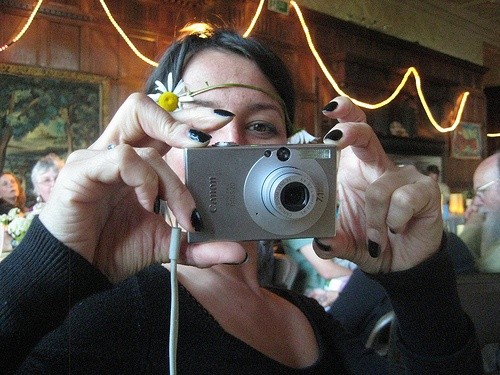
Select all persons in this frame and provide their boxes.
[458,153,500,273]
[0,171,31,215]
[257,238,358,314]
[31,153,65,202]
[0,28,485,375]
[425,165,451,205]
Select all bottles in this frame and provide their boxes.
[465,192,473,208]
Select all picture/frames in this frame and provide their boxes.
[0,63,111,214]
[449,119,485,161]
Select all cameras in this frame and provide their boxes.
[183,143,337,244]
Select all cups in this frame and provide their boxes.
[449,193,464,215]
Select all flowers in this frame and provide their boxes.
[147,72,193,113]
[0,202,45,248]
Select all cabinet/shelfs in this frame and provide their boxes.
[328,48,460,155]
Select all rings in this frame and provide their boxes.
[107,144,115,150]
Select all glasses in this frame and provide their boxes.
[35,175,59,188]
[473,181,495,200]
